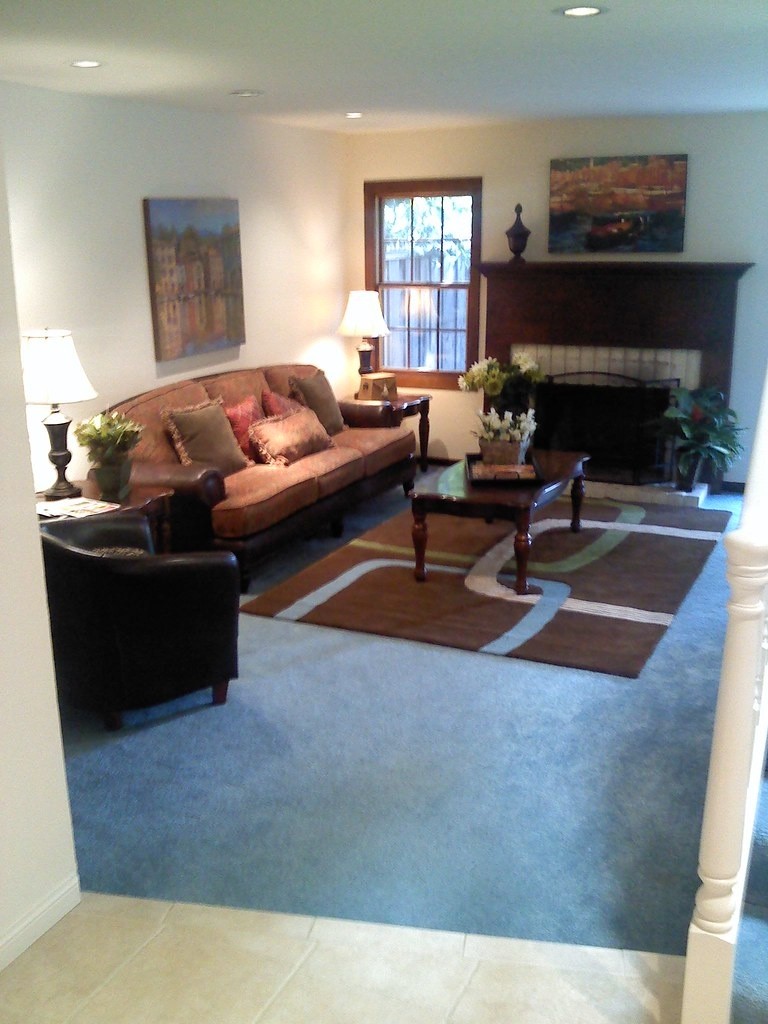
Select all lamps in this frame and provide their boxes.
[338,290,392,399]
[18,330,99,500]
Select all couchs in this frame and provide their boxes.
[96,365,416,593]
[38,513,239,731]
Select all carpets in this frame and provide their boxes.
[238,494,733,680]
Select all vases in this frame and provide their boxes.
[476,437,531,464]
[489,404,530,435]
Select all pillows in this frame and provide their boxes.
[161,370,346,476]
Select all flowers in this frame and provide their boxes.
[473,408,535,439]
[459,345,549,403]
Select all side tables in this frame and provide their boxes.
[354,393,433,474]
[36,479,175,553]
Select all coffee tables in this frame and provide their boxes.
[411,452,597,594]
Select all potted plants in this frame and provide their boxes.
[665,380,750,491]
[80,415,144,499]
